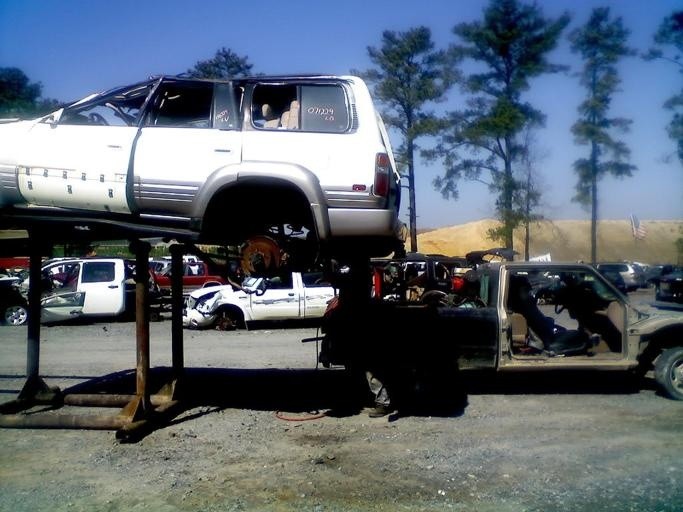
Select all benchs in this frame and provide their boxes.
[629,213,648,242]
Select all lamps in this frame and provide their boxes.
[261,100,299,130]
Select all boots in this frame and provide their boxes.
[1,73,401,278]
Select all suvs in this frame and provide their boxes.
[1,73,401,278]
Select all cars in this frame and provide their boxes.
[595,259,683,305]
[0,253,338,332]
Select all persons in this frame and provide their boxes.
[365,368,394,417]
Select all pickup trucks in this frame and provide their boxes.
[321,261,683,397]
